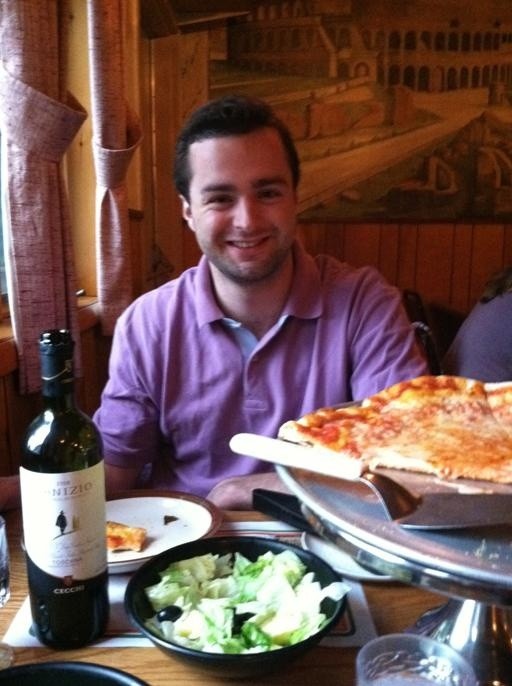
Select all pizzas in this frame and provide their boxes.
[276,375,512,489]
[106,520,146,552]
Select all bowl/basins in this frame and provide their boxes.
[125,537,347,671]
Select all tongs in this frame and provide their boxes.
[252,488,390,576]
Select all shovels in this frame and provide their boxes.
[229,432,512,531]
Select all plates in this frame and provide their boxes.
[274,399,512,586]
[300,530,398,582]
[21,489,222,575]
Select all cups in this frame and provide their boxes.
[355,633,478,686]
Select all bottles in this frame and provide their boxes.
[17,328,111,651]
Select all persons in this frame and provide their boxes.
[437,253,512,383]
[0,95,432,512]
